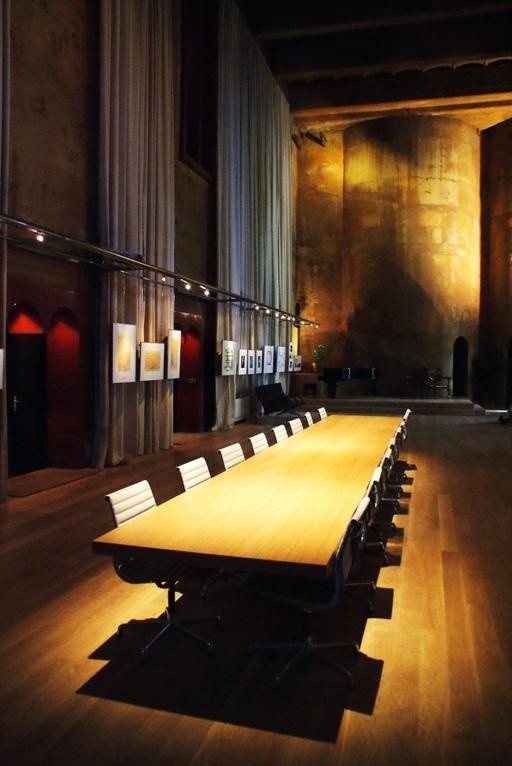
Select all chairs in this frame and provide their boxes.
[424,365,452,399]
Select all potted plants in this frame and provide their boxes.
[311,343,330,374]
